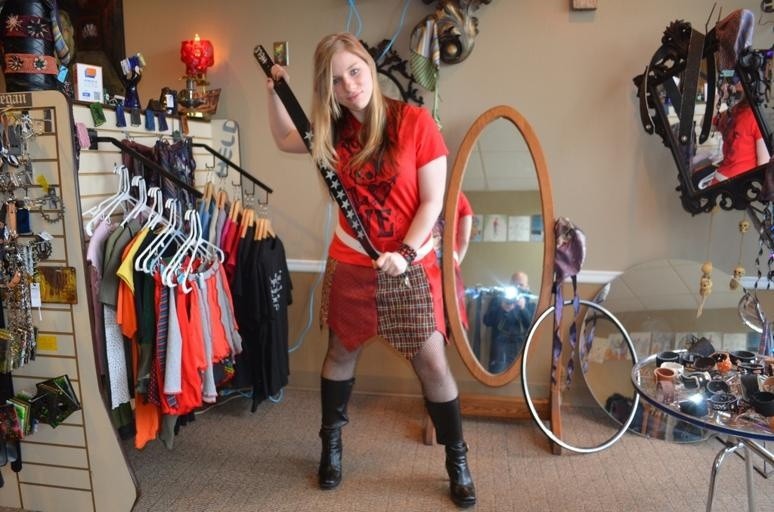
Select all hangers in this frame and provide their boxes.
[79,144,278,294]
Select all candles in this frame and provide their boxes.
[177,33,223,120]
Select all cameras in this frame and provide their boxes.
[503,284,517,306]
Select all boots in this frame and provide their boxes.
[317,377,356,487]
[423,394,476,506]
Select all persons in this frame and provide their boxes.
[703,84,771,185]
[452,190,473,331]
[266,31,476,509]
[482,271,537,374]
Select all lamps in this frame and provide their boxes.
[633,349,774,512]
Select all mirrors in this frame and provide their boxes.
[443,105,554,385]
[579,259,774,444]
